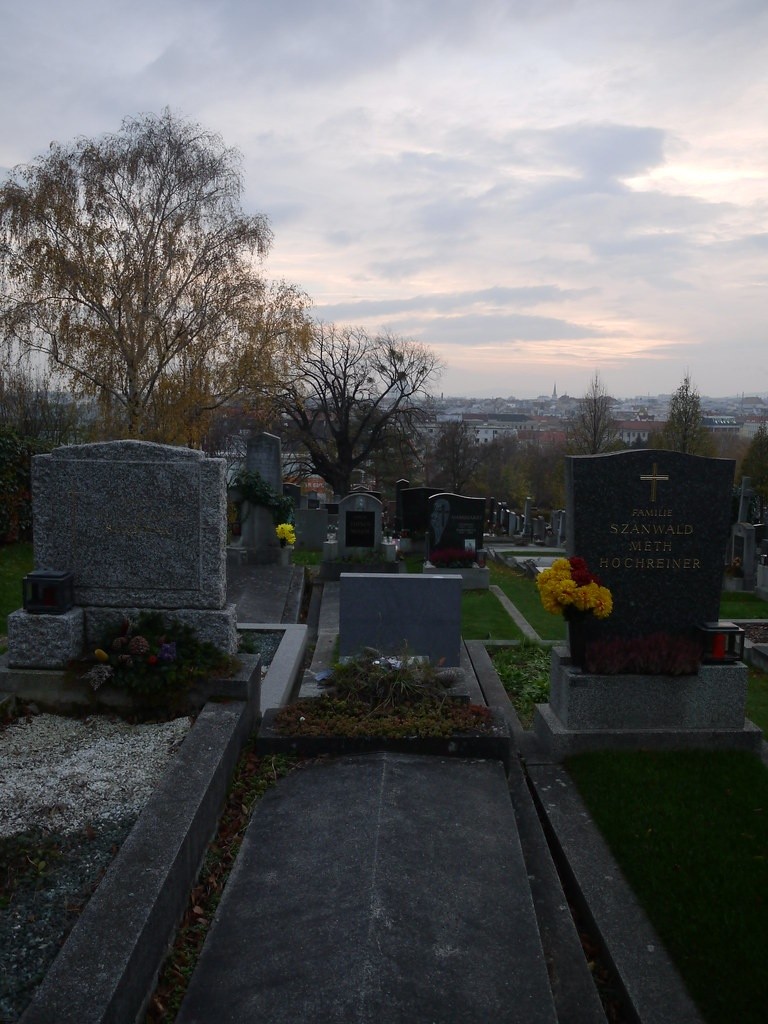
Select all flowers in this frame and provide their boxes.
[429,547,475,568]
[581,631,699,675]
[535,556,613,620]
[275,523,297,544]
[724,557,745,578]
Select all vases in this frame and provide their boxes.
[563,604,597,665]
[280,538,287,549]
